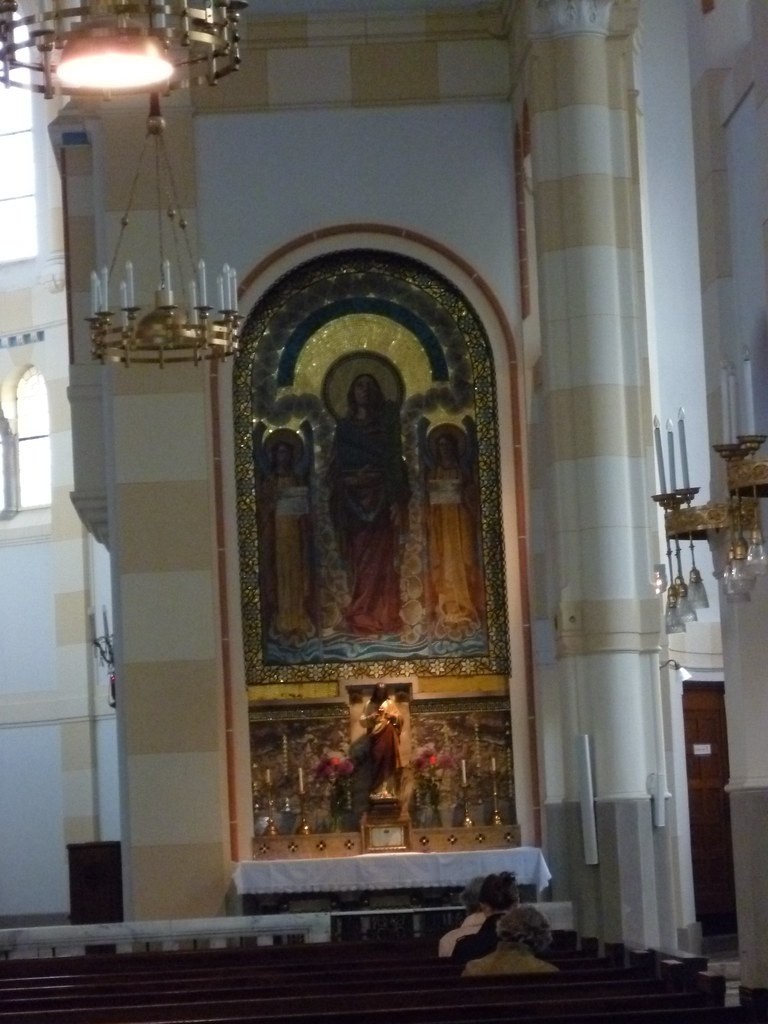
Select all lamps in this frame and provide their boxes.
[86,602,116,709]
[650,346,766,634]
[83,95,245,369]
[660,659,692,682]
[0,1,245,100]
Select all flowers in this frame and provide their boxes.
[414,749,457,827]
[322,751,353,833]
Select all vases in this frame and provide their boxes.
[331,784,351,832]
[417,779,443,827]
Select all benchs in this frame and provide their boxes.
[0,925,768,1024]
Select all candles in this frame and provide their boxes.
[461,760,466,783]
[298,767,305,793]
[492,757,496,771]
[266,768,270,784]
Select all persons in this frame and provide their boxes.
[412,740,448,808]
[437,871,559,978]
[314,745,353,812]
[254,375,486,648]
[359,682,404,799]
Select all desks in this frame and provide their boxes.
[236,846,553,914]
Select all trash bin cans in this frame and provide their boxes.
[65,840,124,925]
